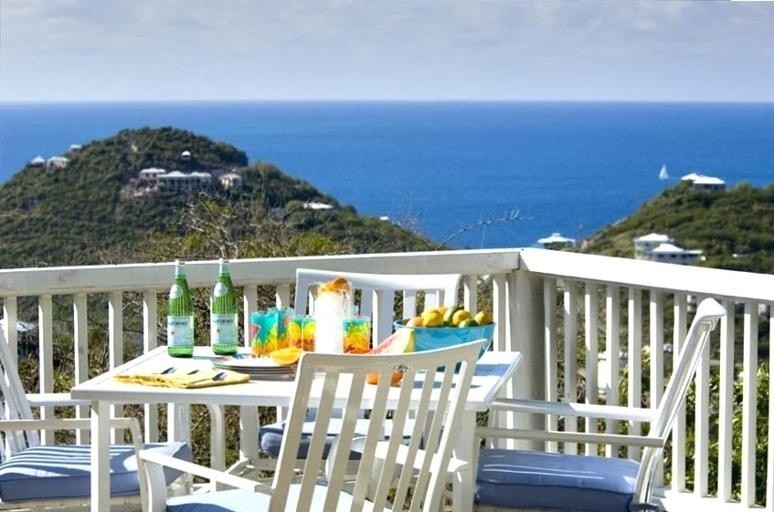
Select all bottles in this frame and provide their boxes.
[166,254,197,359]
[208,253,240,356]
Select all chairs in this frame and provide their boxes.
[0,325,192,512]
[137,340,487,512]
[259,267,461,457]
[476,297,725,512]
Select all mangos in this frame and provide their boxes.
[407,305,487,328]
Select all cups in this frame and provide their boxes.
[249,305,373,364]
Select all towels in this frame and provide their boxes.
[115,367,250,389]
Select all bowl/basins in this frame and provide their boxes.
[390,314,496,373]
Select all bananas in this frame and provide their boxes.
[363,328,415,384]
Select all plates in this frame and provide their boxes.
[207,351,305,376]
